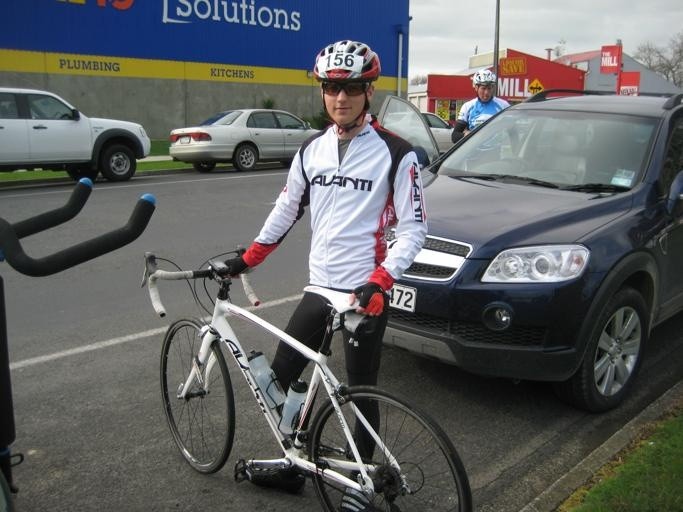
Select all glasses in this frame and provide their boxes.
[318,79,370,96]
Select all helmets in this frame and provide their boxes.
[311,39,383,86]
[472,69,498,88]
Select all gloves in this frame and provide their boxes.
[350,281,390,318]
[223,255,250,280]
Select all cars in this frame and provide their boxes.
[0,88,151,181]
[168,108,324,173]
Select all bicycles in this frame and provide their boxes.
[139,246,474,512]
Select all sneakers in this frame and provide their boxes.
[338,469,373,511]
[245,462,308,496]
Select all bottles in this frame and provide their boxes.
[247,349,287,411]
[277,377,309,435]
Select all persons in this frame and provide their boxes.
[224,40,428,511]
[451,69,519,171]
